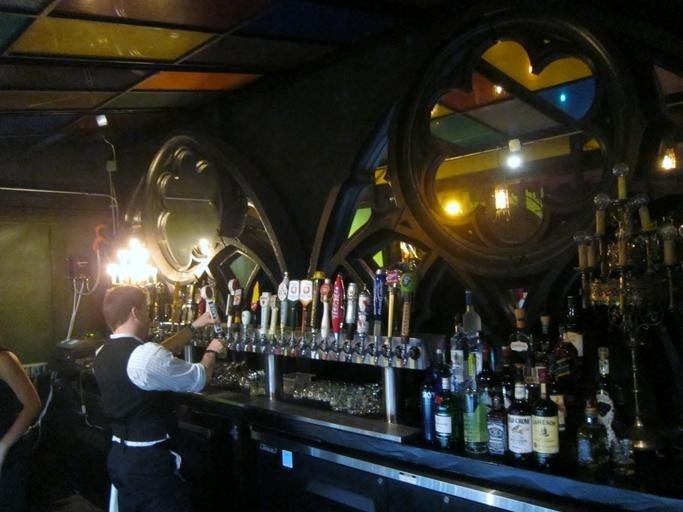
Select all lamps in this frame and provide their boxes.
[492,151,511,218]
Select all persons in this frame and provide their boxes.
[91,284,228,511]
[0,345,43,511]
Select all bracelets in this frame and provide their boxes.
[204,348,219,355]
[188,323,196,334]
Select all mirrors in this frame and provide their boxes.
[150,143,230,274]
[406,11,628,263]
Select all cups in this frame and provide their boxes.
[292,378,385,419]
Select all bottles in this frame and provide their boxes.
[418,287,633,473]
